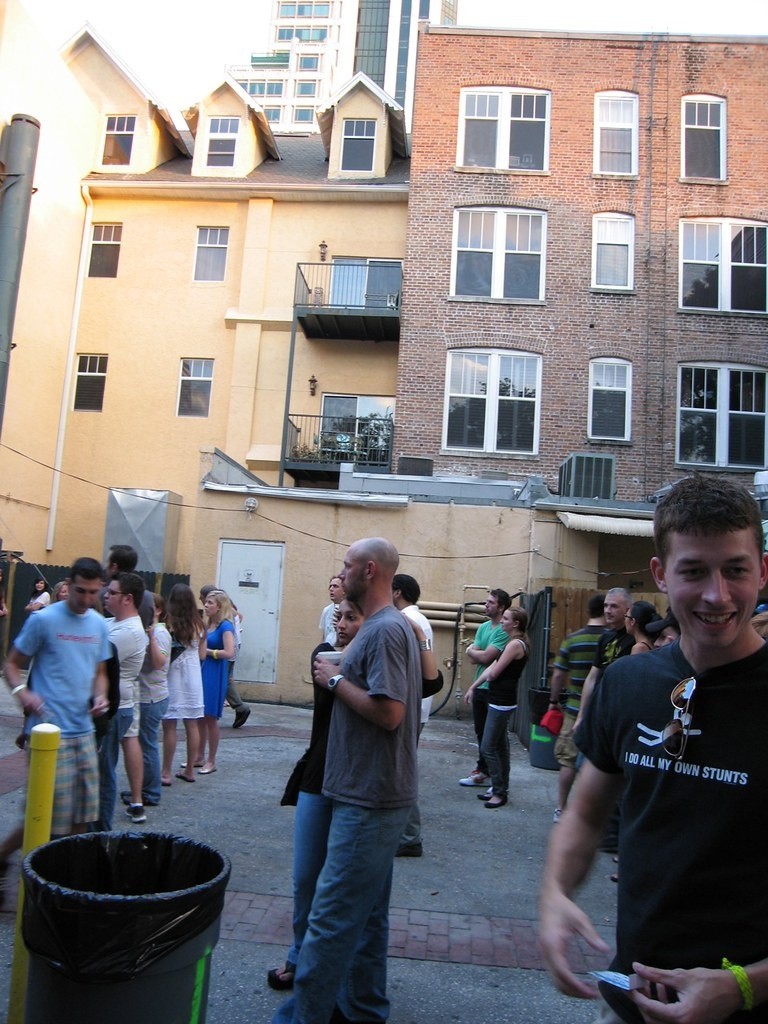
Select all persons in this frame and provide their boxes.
[548,588,768,881]
[0,544,252,914]
[537,475,768,1024]
[460,588,529,810]
[267,536,443,1024]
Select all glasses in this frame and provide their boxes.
[661,676,697,762]
[623,613,632,620]
[107,588,126,595]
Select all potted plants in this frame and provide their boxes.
[290,405,394,466]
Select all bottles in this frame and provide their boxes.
[37,703,55,723]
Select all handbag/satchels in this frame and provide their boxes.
[281,747,312,806]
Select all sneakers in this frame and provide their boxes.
[125,802,147,823]
[233,708,250,728]
[458,772,492,787]
[467,768,482,778]
[552,808,563,824]
[121,790,159,806]
[394,841,422,858]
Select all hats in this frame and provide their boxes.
[754,603,768,614]
[645,612,680,633]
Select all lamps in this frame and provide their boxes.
[307,374,317,396]
[319,240,328,262]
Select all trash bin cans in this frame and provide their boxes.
[528,684,569,771]
[25,832,231,1024]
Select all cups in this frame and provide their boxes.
[318,652,342,666]
[335,604,339,610]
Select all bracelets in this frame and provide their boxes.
[720,955,755,1015]
[10,683,28,696]
[212,648,219,660]
[419,637,433,652]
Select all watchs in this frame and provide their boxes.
[327,674,344,690]
[549,699,558,706]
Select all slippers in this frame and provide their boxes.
[610,872,619,882]
[176,771,195,782]
[161,782,171,786]
[612,856,619,863]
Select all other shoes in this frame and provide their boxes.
[268,962,295,991]
[477,793,508,803]
[484,793,507,808]
[0,861,14,906]
[198,765,217,774]
[181,760,206,768]
